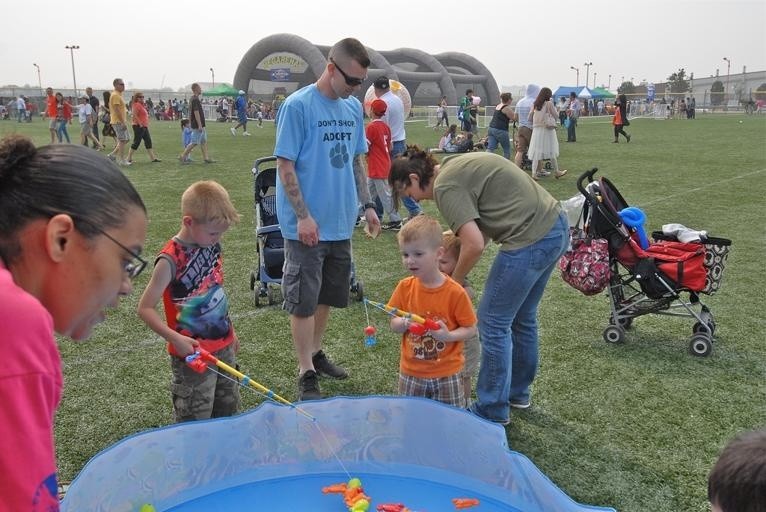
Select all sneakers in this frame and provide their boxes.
[243,132,251,136]
[508,400,531,409]
[230,128,236,137]
[312,348,348,380]
[382,220,403,231]
[298,368,321,403]
[626,134,631,143]
[107,153,120,167]
[537,170,551,177]
[119,159,132,167]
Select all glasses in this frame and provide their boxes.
[119,83,124,85]
[137,96,144,99]
[71,215,148,280]
[329,56,368,86]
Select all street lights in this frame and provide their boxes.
[64,44,81,103]
[620,74,647,84]
[591,72,598,87]
[606,74,612,89]
[583,60,593,89]
[722,56,732,110]
[568,64,580,88]
[208,66,215,87]
[32,62,43,96]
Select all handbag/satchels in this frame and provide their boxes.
[559,198,611,295]
[101,112,110,123]
[86,109,97,125]
[546,112,558,129]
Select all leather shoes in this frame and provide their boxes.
[555,169,567,179]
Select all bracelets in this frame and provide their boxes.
[361,200,378,213]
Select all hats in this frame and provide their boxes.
[373,75,390,90]
[76,93,90,100]
[238,90,245,95]
[365,99,387,116]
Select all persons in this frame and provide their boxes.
[0,138,148,512]
[277,38,383,401]
[1,79,583,181]
[386,216,477,416]
[371,75,426,225]
[437,230,481,410]
[612,94,632,143]
[353,96,403,231]
[705,422,766,512]
[387,144,572,429]
[585,96,696,120]
[137,179,238,425]
[745,98,756,108]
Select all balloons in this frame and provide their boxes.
[619,207,650,250]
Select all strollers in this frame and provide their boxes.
[510,119,554,170]
[250,156,365,307]
[563,167,732,357]
